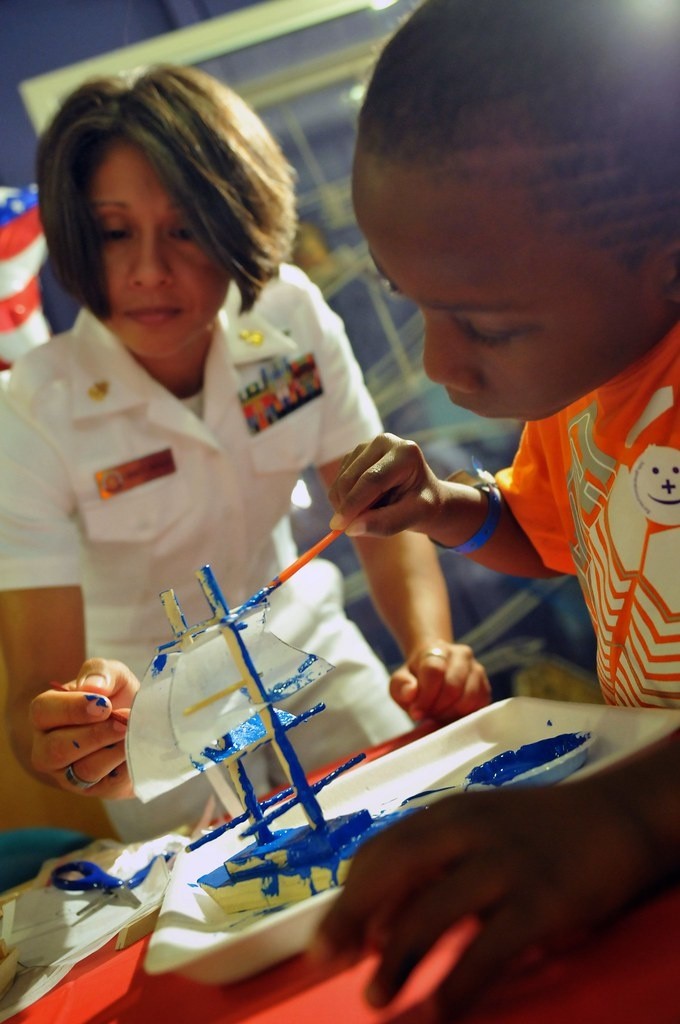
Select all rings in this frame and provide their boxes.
[415,647,446,661]
[64,762,98,789]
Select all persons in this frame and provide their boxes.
[317,0,680,1023]
[0,66,491,846]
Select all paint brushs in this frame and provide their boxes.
[49,680,130,727]
[230,527,344,617]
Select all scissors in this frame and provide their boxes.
[49,852,174,913]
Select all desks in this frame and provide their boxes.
[0,720,680,1024]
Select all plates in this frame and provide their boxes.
[460,730,596,790]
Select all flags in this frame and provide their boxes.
[0,56,79,370]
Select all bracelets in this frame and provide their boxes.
[423,456,502,557]
